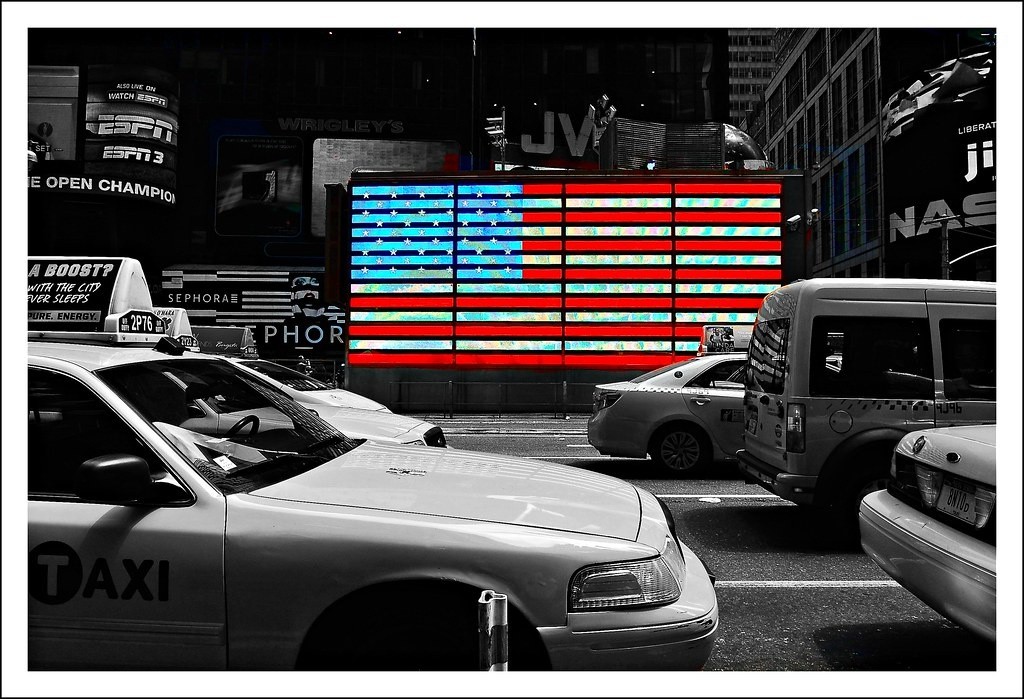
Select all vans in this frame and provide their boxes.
[736,277,996,538]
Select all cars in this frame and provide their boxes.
[859,425,996,643]
[28,339,723,671]
[588,352,752,472]
[222,356,457,454]
